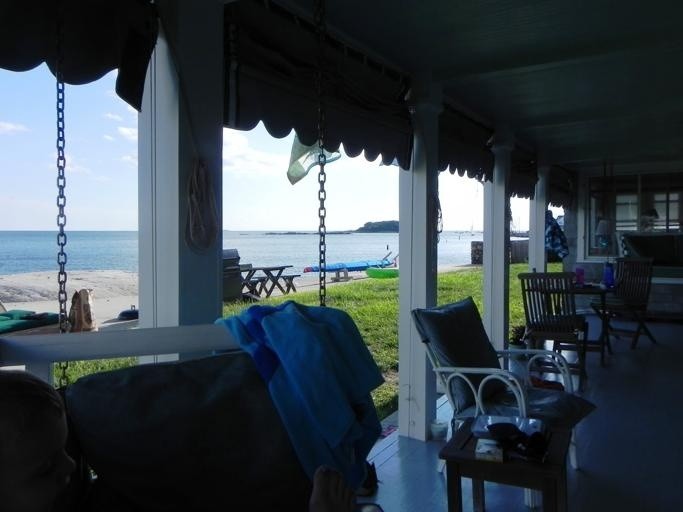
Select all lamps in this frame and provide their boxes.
[640,208,658,231]
[595,219,612,247]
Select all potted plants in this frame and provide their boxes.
[507,325,527,361]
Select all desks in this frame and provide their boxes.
[435,415,569,512]
[522,284,617,362]
[241,258,293,298]
[616,232,683,277]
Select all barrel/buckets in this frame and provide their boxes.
[429,422,449,441]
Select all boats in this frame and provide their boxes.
[365,260,399,278]
[304,259,395,274]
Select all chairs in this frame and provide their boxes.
[410,298,578,509]
[517,271,590,394]
[589,257,657,350]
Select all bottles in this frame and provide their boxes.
[599,262,619,290]
[575,264,584,288]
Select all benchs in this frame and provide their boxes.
[241,276,268,298]
[252,273,302,296]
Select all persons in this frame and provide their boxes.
[0,369,76,511]
[306,462,358,511]
